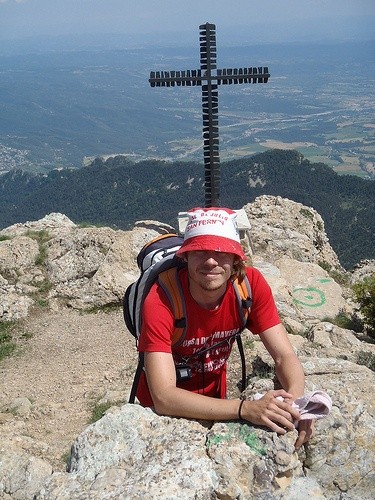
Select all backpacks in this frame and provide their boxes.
[123,234,251,348]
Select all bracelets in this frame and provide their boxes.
[238,399,244,420]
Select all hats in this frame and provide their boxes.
[174,205,248,263]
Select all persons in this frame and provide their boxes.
[128,206,316,451]
